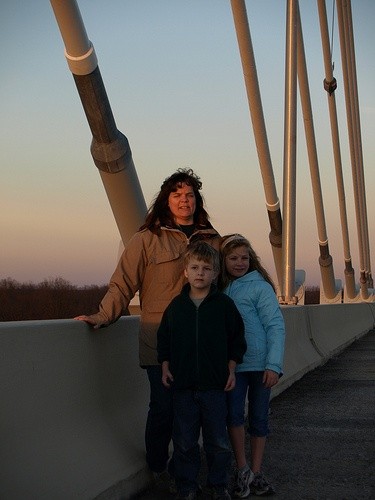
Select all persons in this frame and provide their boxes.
[74,167,226,477]
[219,232,286,500]
[156,246,247,500]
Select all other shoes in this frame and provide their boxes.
[176,487,200,500]
[212,487,231,500]
[145,464,171,489]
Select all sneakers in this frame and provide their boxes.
[249,467,273,496]
[231,465,254,498]
